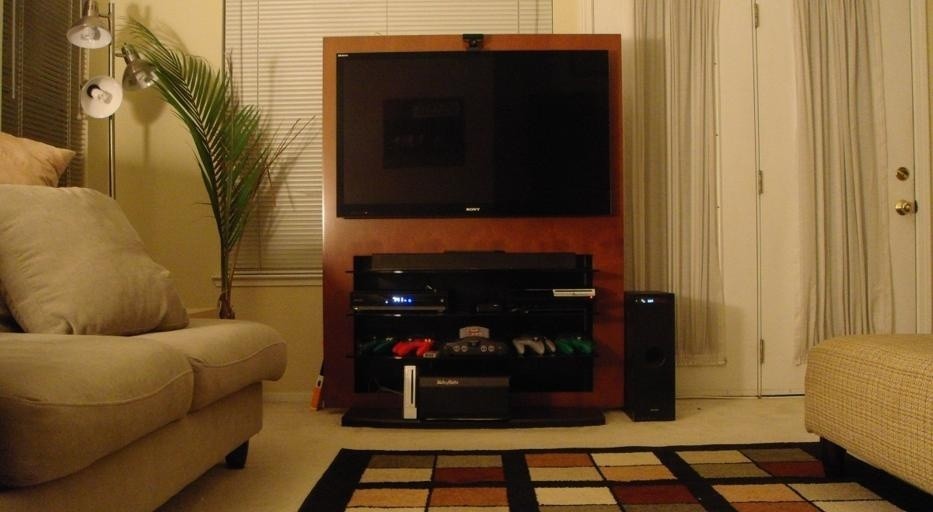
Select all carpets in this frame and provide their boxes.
[293,442,932,510]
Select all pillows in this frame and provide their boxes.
[1,186,189,335]
[1,130,76,188]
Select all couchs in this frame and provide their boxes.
[803,338,933,495]
[1,131,289,510]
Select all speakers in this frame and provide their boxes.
[622,290,676,422]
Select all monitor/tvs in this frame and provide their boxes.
[336,49,611,220]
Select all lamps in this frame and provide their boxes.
[66,0,162,203]
